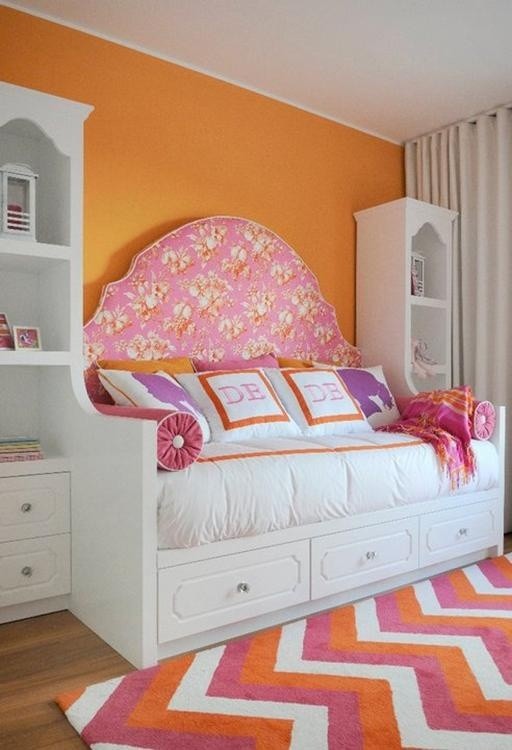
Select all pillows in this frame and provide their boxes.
[97,366,213,442]
[97,404,206,471]
[171,367,301,444]
[309,362,401,429]
[394,393,496,440]
[262,363,376,440]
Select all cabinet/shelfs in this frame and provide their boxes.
[157,495,503,648]
[355,199,456,399]
[1,77,95,630]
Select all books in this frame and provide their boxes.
[0,437,46,462]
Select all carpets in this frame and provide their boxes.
[50,549,510,748]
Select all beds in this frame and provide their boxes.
[78,211,505,671]
